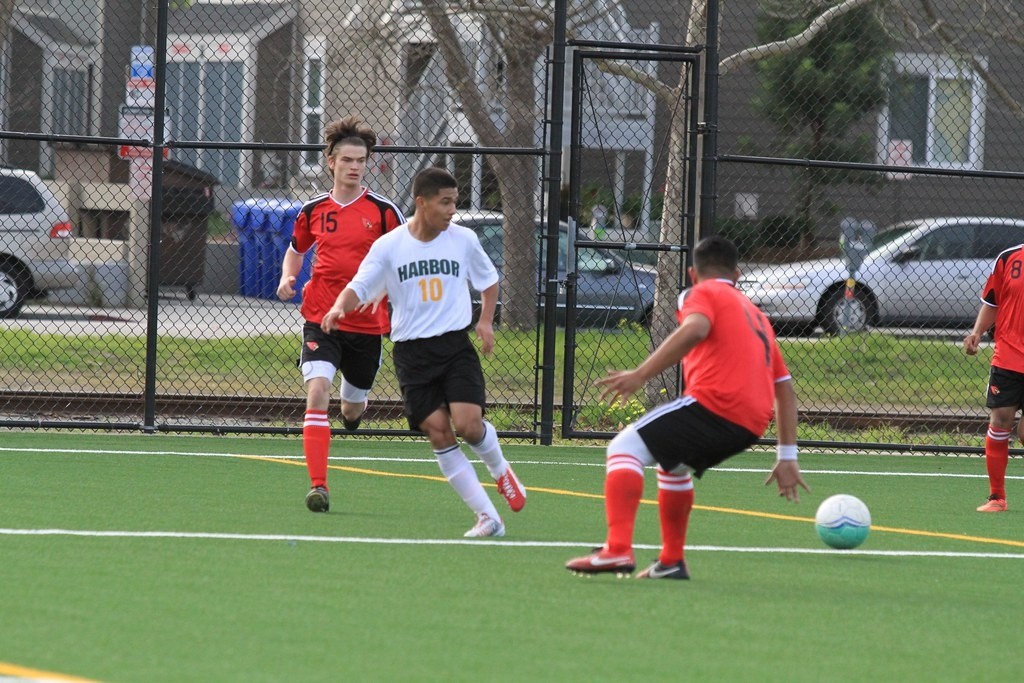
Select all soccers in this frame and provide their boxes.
[815,493,872,550]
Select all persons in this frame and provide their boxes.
[276,116,406,512]
[323,167,527,541]
[964,243,1024,512]
[566,237,809,582]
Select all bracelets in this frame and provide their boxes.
[776,444,800,461]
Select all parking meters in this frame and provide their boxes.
[839,217,876,336]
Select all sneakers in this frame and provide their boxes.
[565,543,634,580]
[306,486,329,512]
[977,494,1007,513]
[491,463,526,512]
[343,414,361,431]
[635,558,690,578]
[464,514,506,538]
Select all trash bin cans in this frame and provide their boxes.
[234,197,316,303]
[163,160,221,298]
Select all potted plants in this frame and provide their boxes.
[581,182,643,230]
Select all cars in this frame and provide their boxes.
[404,209,658,329]
[0,164,80,318]
[733,217,1024,337]
